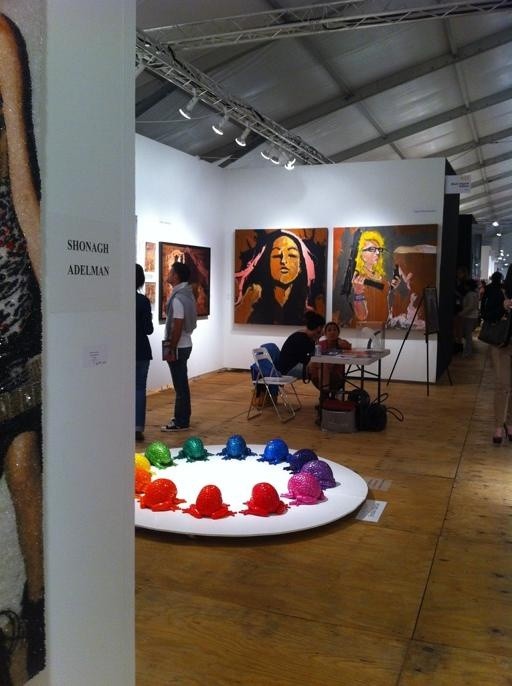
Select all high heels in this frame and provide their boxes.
[493,422,511,446]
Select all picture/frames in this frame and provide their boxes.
[159,241,210,319]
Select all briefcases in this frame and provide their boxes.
[320,398,357,432]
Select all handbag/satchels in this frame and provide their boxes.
[358,403,387,432]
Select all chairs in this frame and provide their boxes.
[248,348,296,423]
[259,343,302,413]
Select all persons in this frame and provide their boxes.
[0,12,44,684]
[278,313,331,404]
[160,261,196,432]
[451,271,505,359]
[348,229,411,327]
[319,321,352,394]
[235,229,323,325]
[135,263,153,442]
[487,262,512,444]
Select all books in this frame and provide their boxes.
[162,339,179,360]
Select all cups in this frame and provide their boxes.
[314,344,321,357]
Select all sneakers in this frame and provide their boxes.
[137,432,146,442]
[19,578,47,671]
[160,420,192,432]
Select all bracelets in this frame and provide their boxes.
[352,292,366,302]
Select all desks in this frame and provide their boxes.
[311,349,390,425]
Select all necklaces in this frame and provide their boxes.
[363,264,377,274]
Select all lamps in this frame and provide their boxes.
[179,92,297,170]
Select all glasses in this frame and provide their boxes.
[362,247,384,253]
